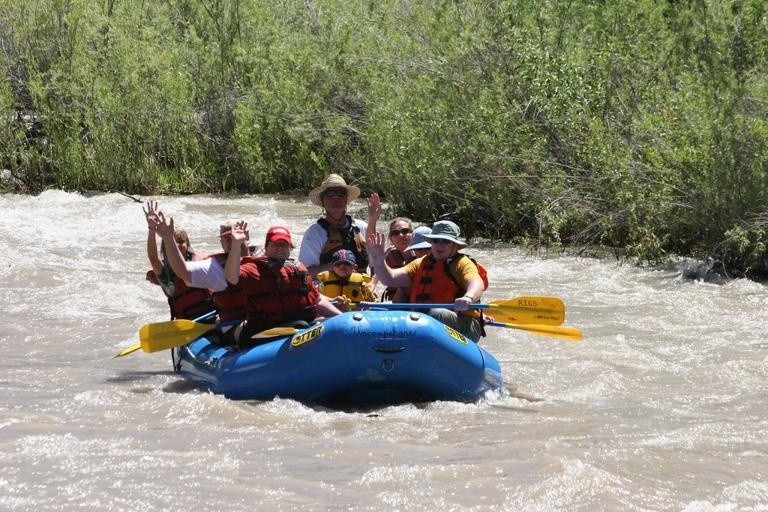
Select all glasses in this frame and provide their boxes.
[335,261,349,266]
[433,239,451,244]
[325,190,346,196]
[391,228,413,236]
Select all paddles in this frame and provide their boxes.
[114,309,215,356]
[482,326,583,339]
[138,297,351,354]
[478,301,520,324]
[346,296,564,324]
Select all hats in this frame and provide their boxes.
[422,220,468,250]
[309,174,360,206]
[267,226,294,248]
[332,249,356,266]
[403,226,432,253]
[220,225,232,238]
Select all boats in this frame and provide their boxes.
[176,304,503,407]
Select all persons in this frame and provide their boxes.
[390,225,433,304]
[366,191,416,303]
[298,173,373,282]
[316,249,376,315]
[147,209,269,334]
[141,199,213,335]
[368,221,484,345]
[225,218,342,338]
